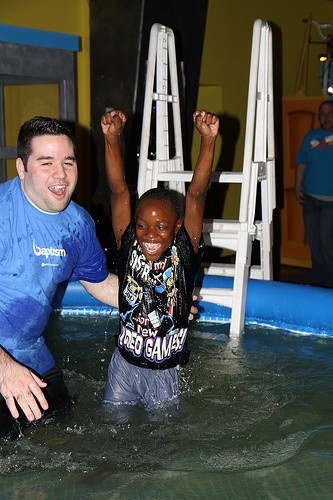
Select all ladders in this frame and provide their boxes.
[139,18,277,343]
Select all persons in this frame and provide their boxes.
[101,108,220,404]
[0,115,197,444]
[295,100,333,288]
